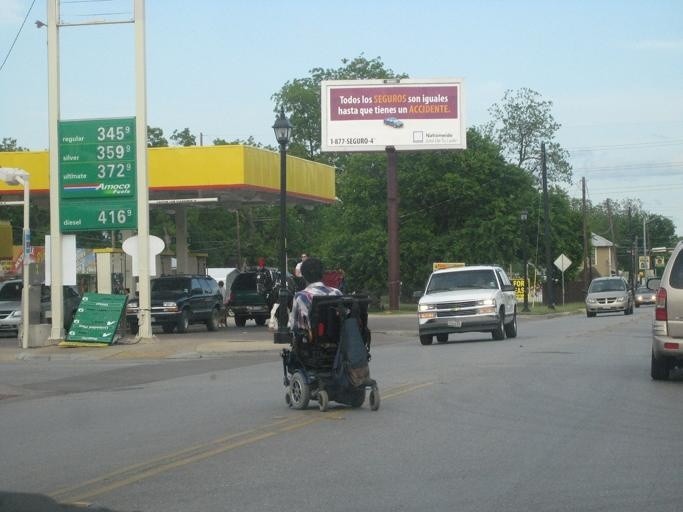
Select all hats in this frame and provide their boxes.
[301,258,324,283]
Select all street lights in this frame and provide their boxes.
[272,101,296,336]
[520,207,532,312]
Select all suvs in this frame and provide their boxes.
[227,267,298,327]
[124,272,226,336]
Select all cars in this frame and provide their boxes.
[0,279,83,339]
[585,275,633,318]
[633,284,657,308]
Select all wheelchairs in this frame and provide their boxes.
[280,294,381,413]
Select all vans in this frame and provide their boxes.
[417,266,518,345]
[651,233,683,382]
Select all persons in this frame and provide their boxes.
[218,280,226,328]
[288,258,344,343]
[294,253,307,277]
